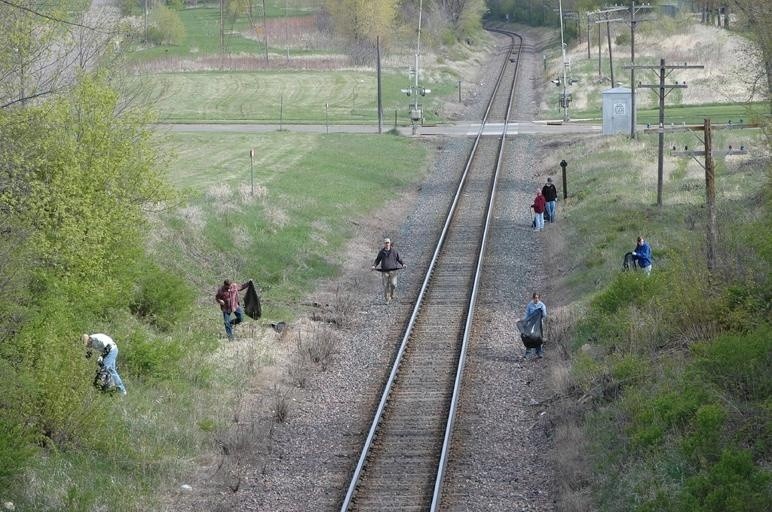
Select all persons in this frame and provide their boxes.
[216,278,253,342]
[532,188,546,232]
[80,332,128,397]
[541,177,557,223]
[523,292,548,359]
[631,236,653,279]
[371,238,407,305]
